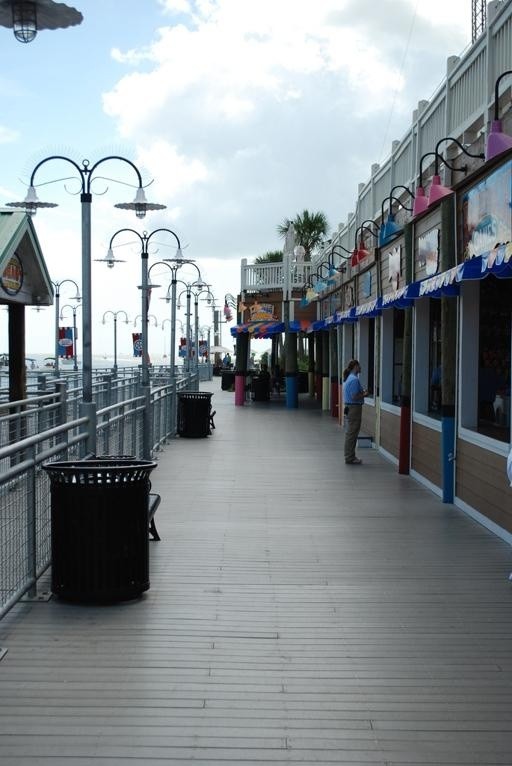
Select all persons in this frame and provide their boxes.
[343,359,369,463]
[222,351,268,370]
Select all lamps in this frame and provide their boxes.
[298,185,422,307]
[411,70,512,217]
[222,292,237,322]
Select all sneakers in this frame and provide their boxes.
[346,457,363,465]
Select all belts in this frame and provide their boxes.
[344,403,363,405]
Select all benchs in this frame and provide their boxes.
[209,404,216,429]
[79,453,161,541]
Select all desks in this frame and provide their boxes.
[213,362,285,401]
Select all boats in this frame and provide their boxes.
[1,352,82,378]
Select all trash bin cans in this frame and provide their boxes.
[220,370,236,392]
[41,456,158,609]
[175,391,214,440]
